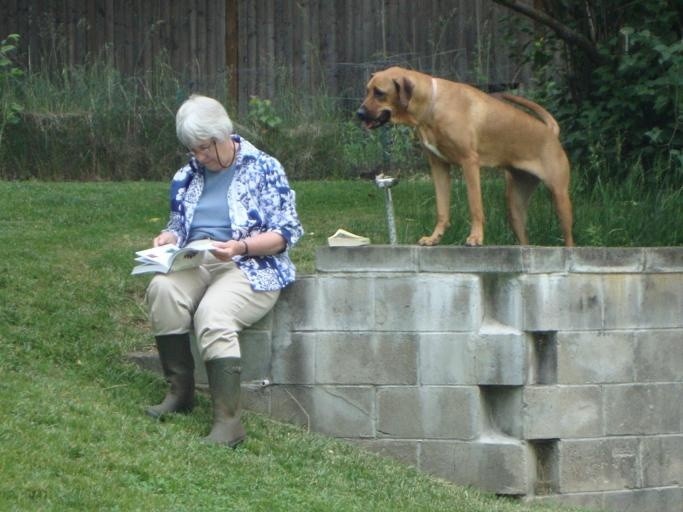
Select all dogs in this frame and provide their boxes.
[355,64,577,249]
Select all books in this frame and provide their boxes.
[132,239,225,276]
[328,229,370,247]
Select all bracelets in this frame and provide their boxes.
[240,240,248,255]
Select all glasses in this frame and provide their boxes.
[186,140,213,157]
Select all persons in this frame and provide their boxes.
[139,95,305,448]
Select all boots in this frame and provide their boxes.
[143,334,195,422]
[199,356,245,448]
